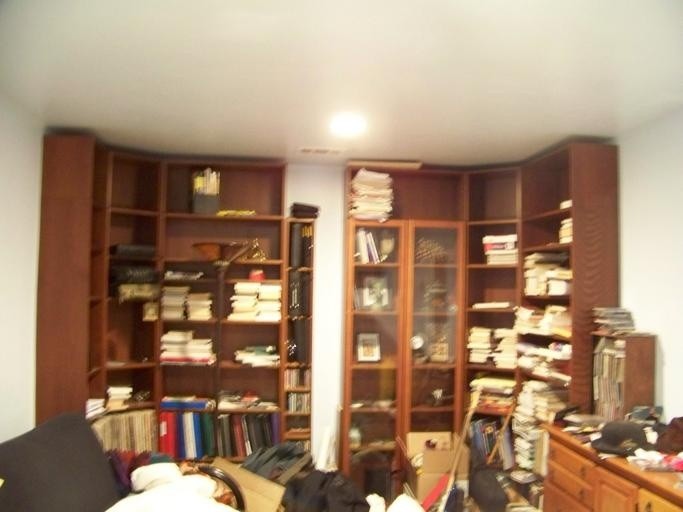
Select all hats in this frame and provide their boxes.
[591,419,654,457]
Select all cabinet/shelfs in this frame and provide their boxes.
[282,221,312,463]
[545,424,680,512]
[463,161,604,505]
[44,133,158,410]
[349,165,461,490]
[160,165,288,470]
[592,328,651,417]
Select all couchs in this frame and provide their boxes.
[0,411,244,512]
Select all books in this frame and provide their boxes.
[589,307,633,419]
[466,199,574,483]
[86,201,313,460]
[351,168,396,309]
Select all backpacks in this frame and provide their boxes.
[239,441,314,501]
[283,469,370,511]
